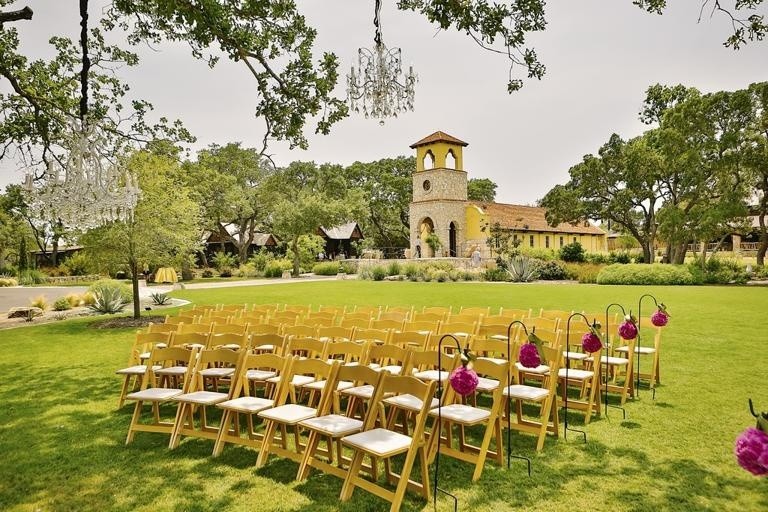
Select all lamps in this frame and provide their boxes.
[18,0,140,233]
[347,0,416,124]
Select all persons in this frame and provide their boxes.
[471,246,481,268]
[413,235,421,258]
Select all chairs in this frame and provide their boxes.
[113,301,669,511]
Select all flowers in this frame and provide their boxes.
[732,414,767,479]
